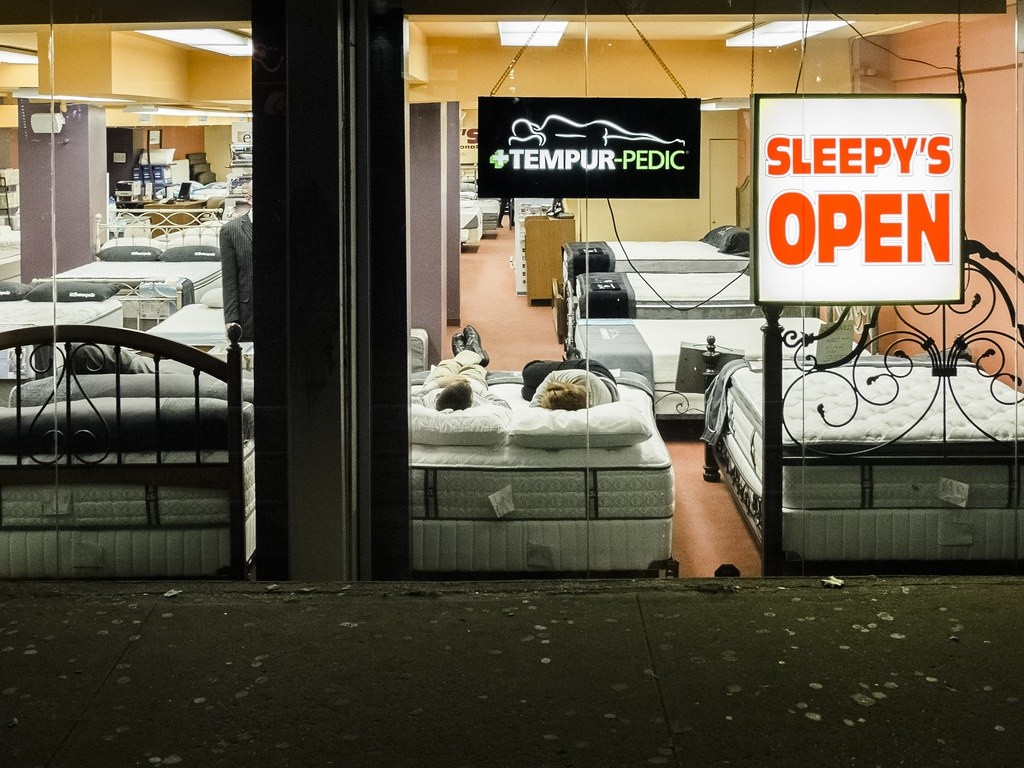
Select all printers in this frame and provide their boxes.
[114,180,142,196]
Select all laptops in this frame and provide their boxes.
[175,182,191,201]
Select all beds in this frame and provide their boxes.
[576,274,766,319]
[574,306,878,419]
[411,371,675,570]
[0,325,255,577]
[561,241,752,296]
[704,230,1024,575]
[460,200,500,230]
[143,304,257,354]
[0,299,123,379]
[36,262,221,319]
[460,209,482,244]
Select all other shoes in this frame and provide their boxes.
[497,222,503,228]
[566,349,581,360]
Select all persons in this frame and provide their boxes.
[219,180,253,343]
[498,197,515,227]
[521,359,621,412]
[419,325,511,412]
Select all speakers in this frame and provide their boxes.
[30,112,66,133]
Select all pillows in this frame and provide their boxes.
[201,288,223,308]
[24,280,121,302]
[157,245,226,262]
[0,282,33,301]
[699,225,749,252]
[410,401,508,446]
[509,403,652,447]
[95,246,164,261]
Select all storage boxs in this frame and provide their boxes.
[133,166,172,197]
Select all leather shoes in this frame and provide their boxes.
[463,324,489,368]
[452,333,466,356]
